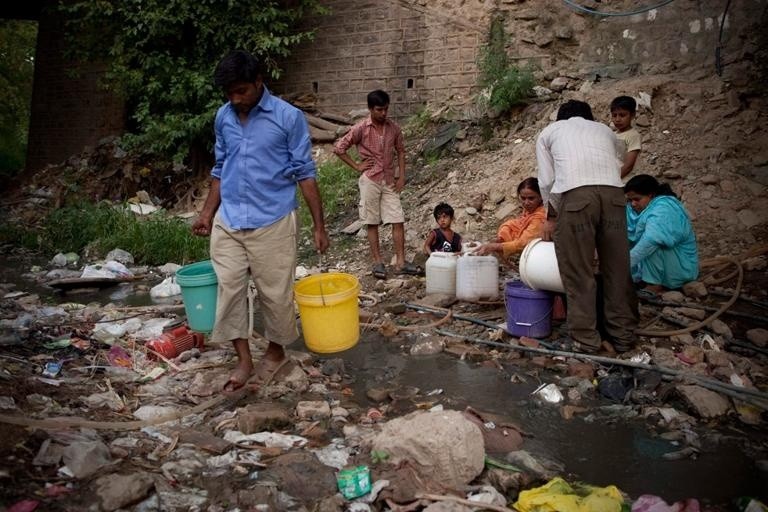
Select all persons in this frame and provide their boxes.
[606,96,644,180]
[470,175,553,261]
[334,88,425,281]
[190,47,333,395]
[422,202,462,257]
[619,173,699,303]
[534,100,641,354]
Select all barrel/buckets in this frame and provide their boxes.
[423,250,455,301]
[455,253,499,306]
[290,270,363,356]
[175,259,217,334]
[516,236,566,294]
[503,278,555,340]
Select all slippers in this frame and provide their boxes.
[223,367,255,395]
[613,342,630,350]
[636,287,663,299]
[551,338,592,352]
[394,262,422,275]
[255,355,290,375]
[372,263,386,279]
